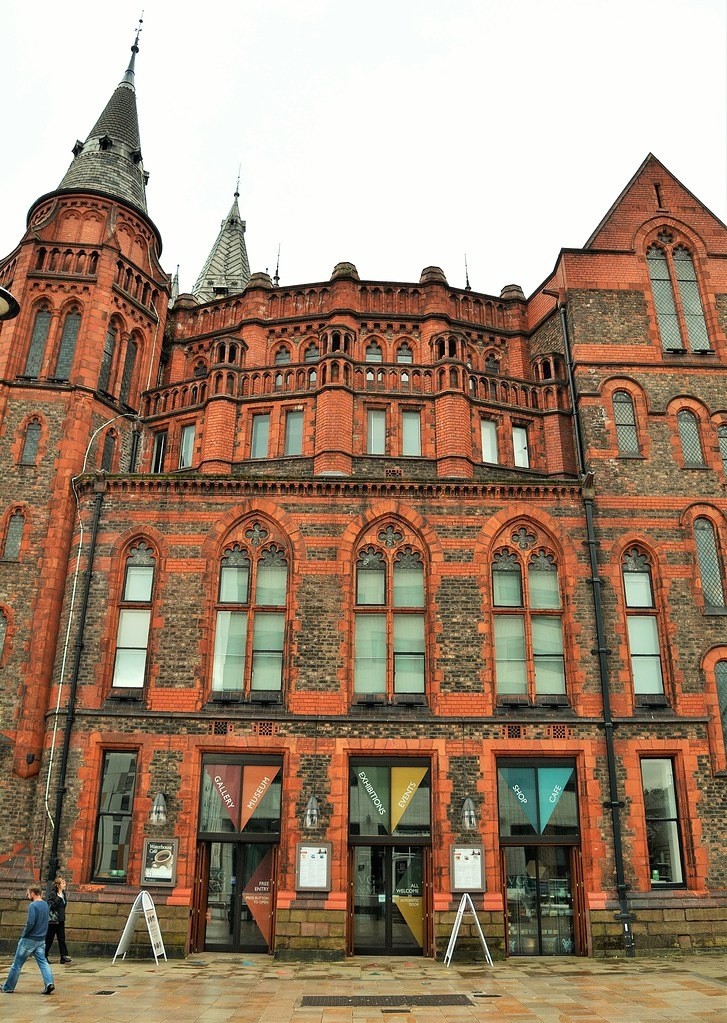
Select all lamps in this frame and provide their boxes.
[148,793,168,825]
[303,793,322,830]
[461,796,477,833]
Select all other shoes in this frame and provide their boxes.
[42,984,55,995]
[46,958,52,964]
[60,956,71,964]
[0,984,13,993]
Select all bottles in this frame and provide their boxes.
[661,852,665,862]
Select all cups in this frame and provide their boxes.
[111,869,118,876]
[653,870,659,881]
[118,870,125,877]
[151,850,171,868]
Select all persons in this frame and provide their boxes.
[46,877,72,964]
[0,885,55,994]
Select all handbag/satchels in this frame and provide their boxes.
[47,904,59,924]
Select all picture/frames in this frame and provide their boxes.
[140,838,179,887]
[450,845,486,893]
[295,843,332,892]
